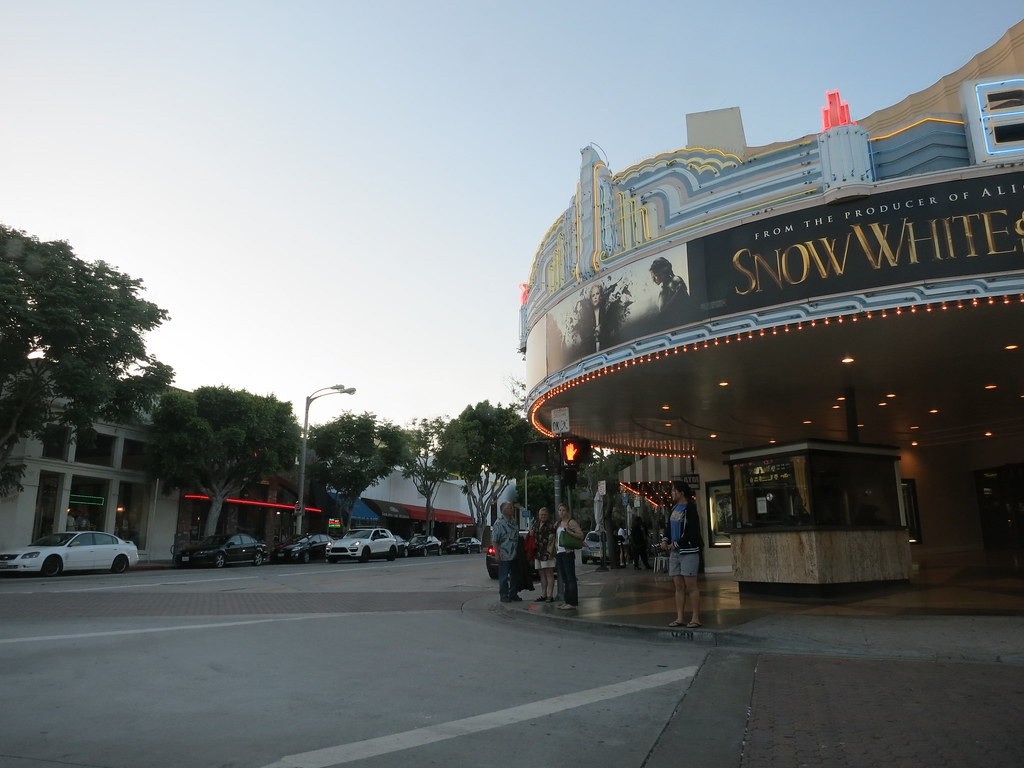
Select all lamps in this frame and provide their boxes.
[114,493,127,511]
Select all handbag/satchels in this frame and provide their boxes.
[559,519,582,549]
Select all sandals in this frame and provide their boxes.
[534,596,546,601]
[546,596,553,603]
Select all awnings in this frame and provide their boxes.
[403,504,476,524]
[324,492,381,521]
[361,497,410,518]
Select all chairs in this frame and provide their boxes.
[751,482,793,526]
[653,537,670,574]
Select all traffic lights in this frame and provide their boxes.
[522,440,551,468]
[562,438,592,465]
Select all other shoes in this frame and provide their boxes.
[509,594,522,601]
[646,565,653,569]
[634,567,641,570]
[500,596,512,602]
[556,603,567,609]
[561,604,576,609]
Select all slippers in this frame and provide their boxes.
[668,621,686,627]
[686,622,702,628]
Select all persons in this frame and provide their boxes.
[578,285,618,358]
[67,509,90,531]
[617,522,631,568]
[552,503,584,610]
[529,506,555,603]
[630,516,653,570]
[491,501,522,602]
[650,257,688,311]
[661,481,703,628]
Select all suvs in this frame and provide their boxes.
[325,527,398,563]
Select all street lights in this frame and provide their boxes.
[294,384,356,533]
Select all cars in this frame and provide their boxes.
[408,535,443,556]
[581,531,610,565]
[446,537,482,554]
[393,535,410,558]
[272,533,334,563]
[485,531,529,578]
[0,531,140,577]
[174,532,268,568]
